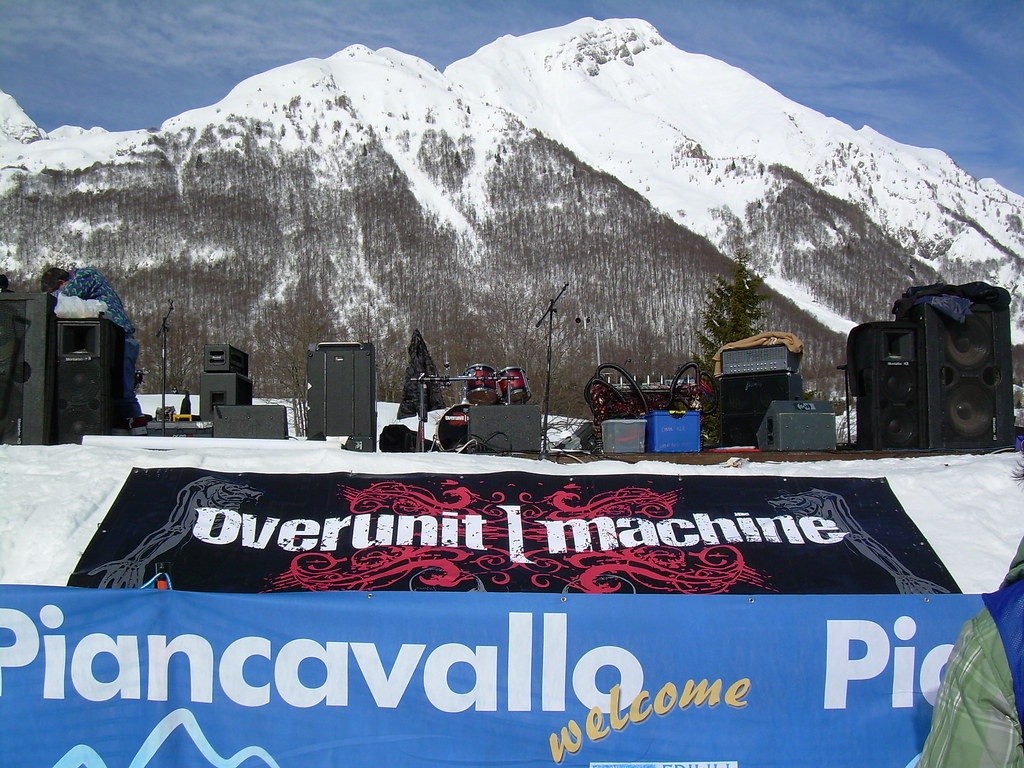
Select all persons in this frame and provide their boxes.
[919,537,1024,768]
[0,274,15,293]
[41,268,144,418]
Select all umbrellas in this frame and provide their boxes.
[396,328,447,451]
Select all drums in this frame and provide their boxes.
[497,366,532,403]
[438,404,472,452]
[463,363,498,406]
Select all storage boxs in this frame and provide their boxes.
[639,412,701,453]
[145,421,213,438]
[602,420,647,453]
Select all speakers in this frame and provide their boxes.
[199,343,289,440]
[47,319,127,446]
[719,372,837,452]
[468,406,542,454]
[845,303,1016,451]
